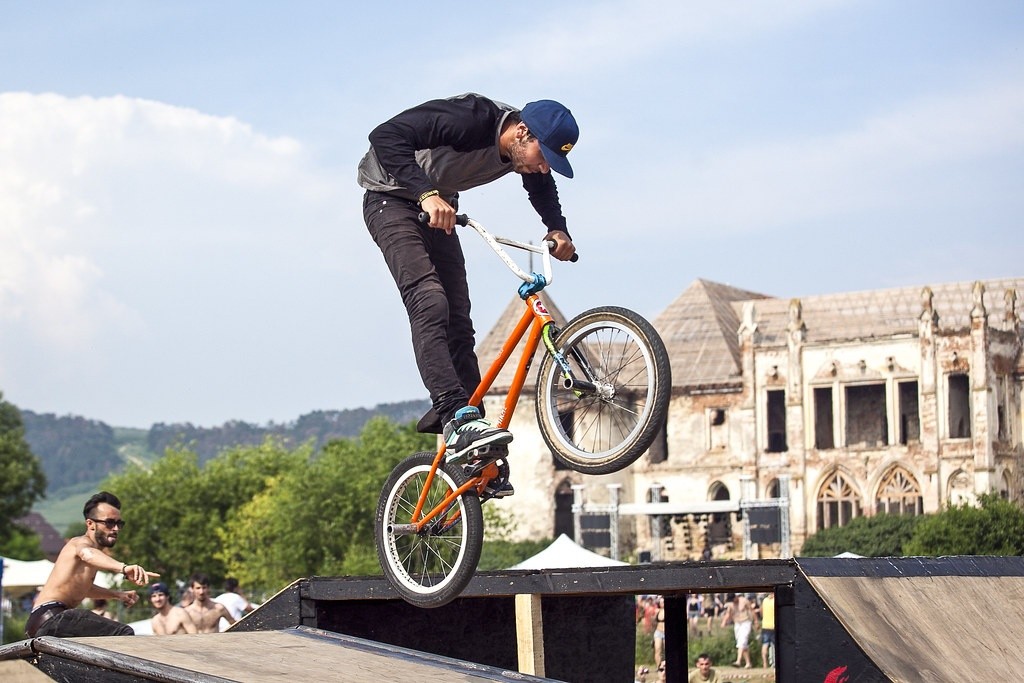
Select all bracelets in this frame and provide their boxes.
[122,565,127,575]
[418,189,439,203]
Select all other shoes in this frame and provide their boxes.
[732,656,770,670]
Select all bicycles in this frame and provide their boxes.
[373,211,674,608]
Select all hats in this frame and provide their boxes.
[147,583,170,599]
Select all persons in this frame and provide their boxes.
[89,598,112,621]
[686,594,703,639]
[701,594,716,638]
[721,593,759,669]
[175,585,196,609]
[148,582,199,636]
[652,598,665,669]
[635,665,648,683]
[636,594,760,632]
[182,575,236,633]
[355,90,577,500]
[689,654,723,683]
[761,593,774,668]
[212,577,254,633]
[23,491,162,640]
[655,661,666,683]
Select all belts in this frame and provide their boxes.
[25,607,66,638]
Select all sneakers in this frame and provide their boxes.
[521,99,580,179]
[441,404,514,463]
[479,472,517,500]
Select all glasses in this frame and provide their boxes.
[86,515,126,531]
